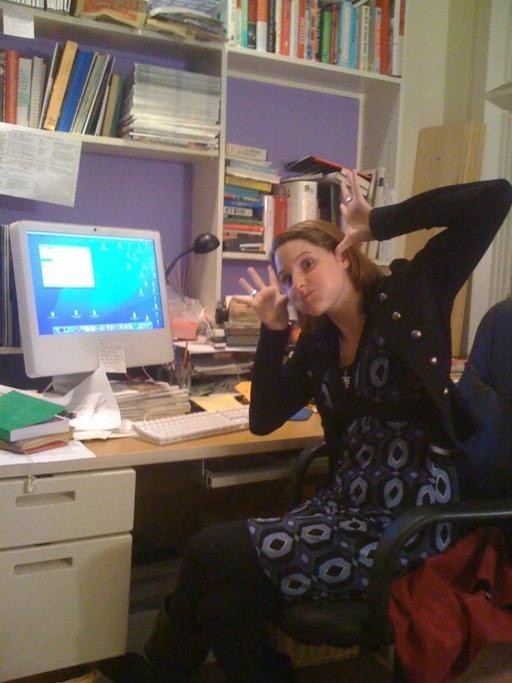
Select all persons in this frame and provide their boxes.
[94,166,512,680]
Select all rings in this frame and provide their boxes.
[343,196,352,205]
[251,289,257,296]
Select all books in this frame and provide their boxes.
[224,140,390,265]
[1,426,74,455]
[224,292,262,346]
[0,34,222,150]
[109,377,192,421]
[231,1,402,78]
[1,390,71,443]
[10,1,226,41]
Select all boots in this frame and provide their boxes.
[96,595,213,683]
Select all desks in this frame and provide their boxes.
[0,391,326,683]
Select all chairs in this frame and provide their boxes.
[271,296,512,683]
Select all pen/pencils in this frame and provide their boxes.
[169,336,190,389]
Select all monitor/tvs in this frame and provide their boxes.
[9,219,175,396]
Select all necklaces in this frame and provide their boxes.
[337,312,367,388]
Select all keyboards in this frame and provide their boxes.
[131,403,250,445]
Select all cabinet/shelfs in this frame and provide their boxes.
[0,0,402,355]
